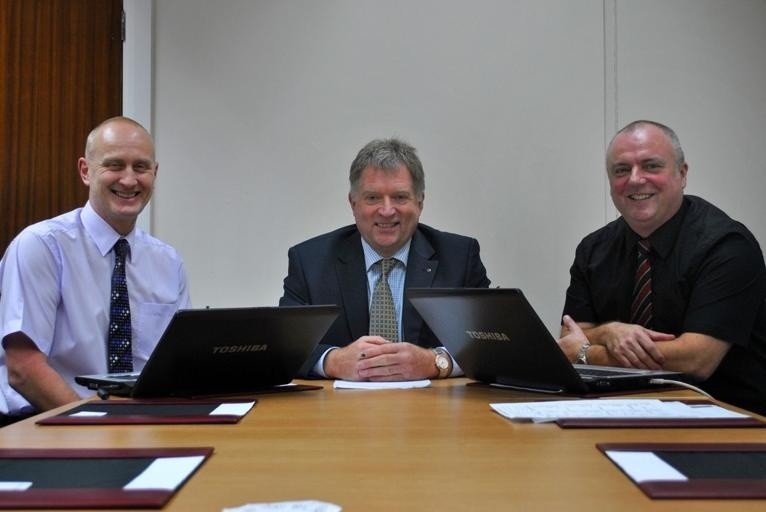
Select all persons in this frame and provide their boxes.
[277,136,491,382]
[555,118,766,420]
[0,115,193,430]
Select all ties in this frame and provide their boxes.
[629,239,656,328]
[365,257,399,344]
[103,238,136,374]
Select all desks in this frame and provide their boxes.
[0,376,766,511]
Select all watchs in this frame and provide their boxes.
[430,345,453,380]
[576,341,591,364]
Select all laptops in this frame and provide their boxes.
[74,304,343,401]
[405,287,686,397]
